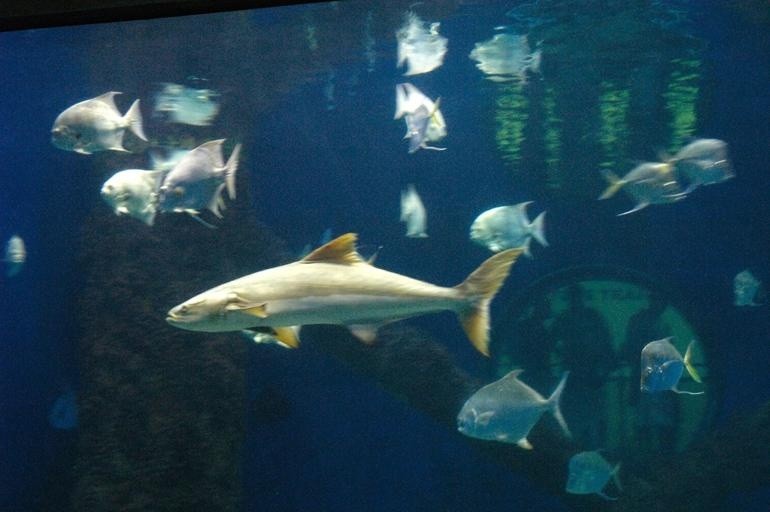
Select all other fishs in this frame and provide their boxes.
[165,232,530,358]
